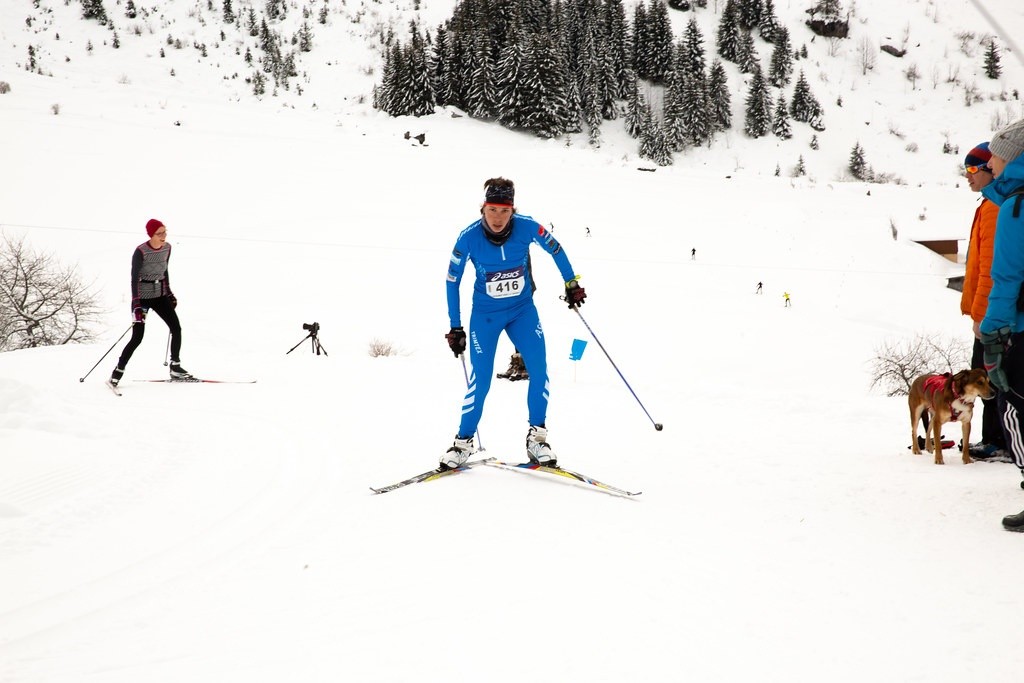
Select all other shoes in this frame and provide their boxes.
[1002,510,1024,533]
[497,354,527,381]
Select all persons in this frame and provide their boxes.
[756,281,763,294]
[110,219,193,387]
[691,248,696,259]
[439,178,587,470]
[979,119,1024,531]
[783,291,790,307]
[960,142,1013,462]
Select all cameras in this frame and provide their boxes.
[303,322,320,331]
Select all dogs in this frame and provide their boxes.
[908,369,996,464]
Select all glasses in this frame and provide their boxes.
[155,231,166,237]
[965,163,986,173]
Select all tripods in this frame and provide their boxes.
[286,330,327,356]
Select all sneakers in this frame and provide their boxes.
[111,368,124,385]
[439,434,475,469]
[527,426,557,465]
[968,441,1012,462]
[170,362,187,378]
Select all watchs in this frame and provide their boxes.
[566,279,578,289]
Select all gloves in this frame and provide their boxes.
[564,274,587,309]
[979,325,1012,392]
[134,305,143,321]
[168,294,177,308]
[445,327,466,357]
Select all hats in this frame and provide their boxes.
[987,118,1024,161]
[146,219,163,237]
[964,141,992,173]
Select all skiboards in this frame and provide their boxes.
[105,359,256,396]
[368,424,643,497]
